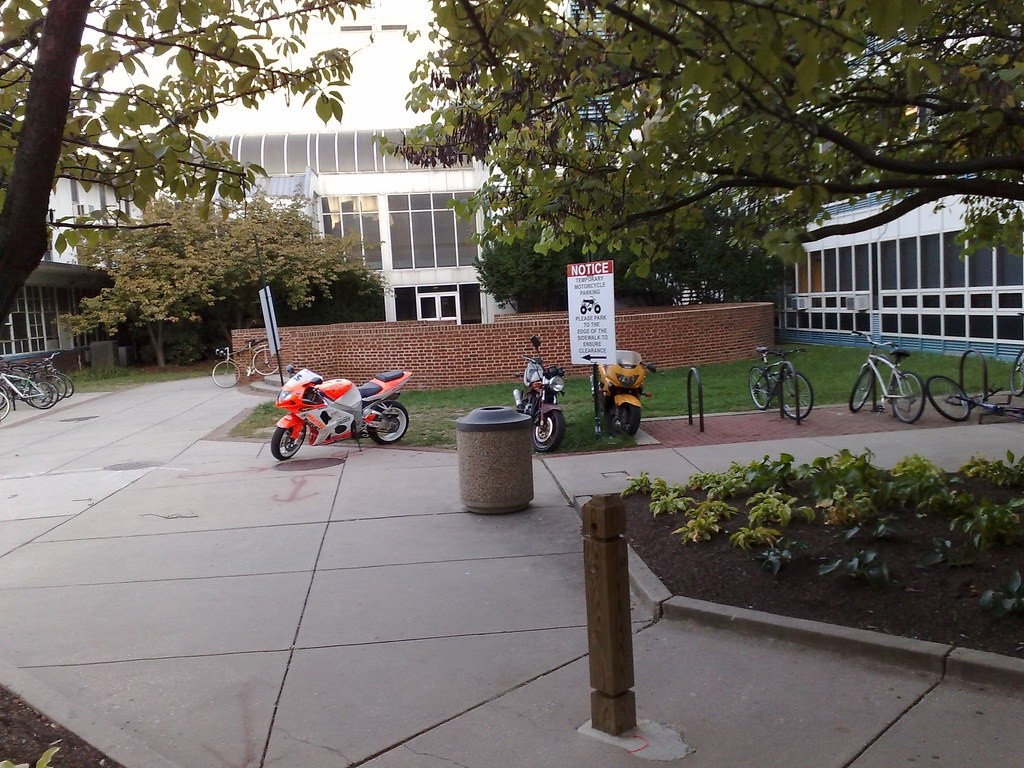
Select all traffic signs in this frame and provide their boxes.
[567,261,617,365]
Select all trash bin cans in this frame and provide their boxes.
[456,407,533,515]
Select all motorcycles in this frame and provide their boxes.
[589,349,657,438]
[513,334,567,453]
[270,363,414,461]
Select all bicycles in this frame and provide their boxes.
[747,346,814,421]
[1009,312,1024,396]
[848,330,928,425]
[924,374,1024,425]
[0,351,74,421]
[212,335,284,389]
